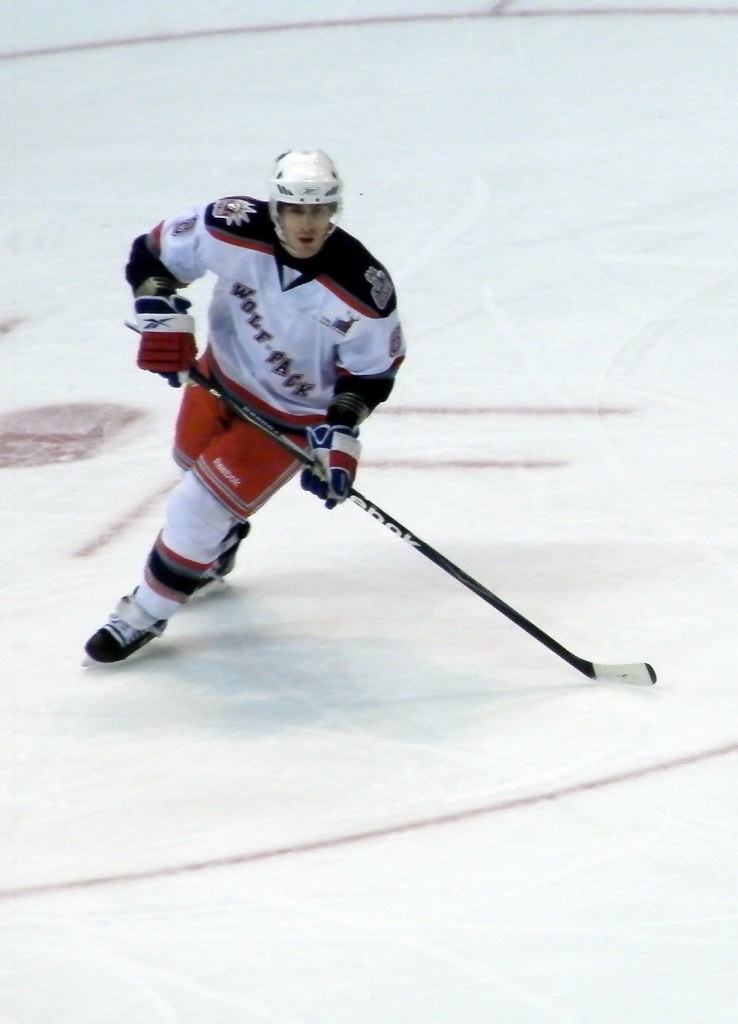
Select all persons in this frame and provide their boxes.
[82,148,406,664]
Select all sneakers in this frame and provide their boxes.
[195,517,250,590]
[80,586,167,668]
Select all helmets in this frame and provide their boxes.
[270,147,344,226]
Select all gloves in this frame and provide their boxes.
[300,432,360,511]
[133,293,197,373]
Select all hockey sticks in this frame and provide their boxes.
[121,313,659,691]
